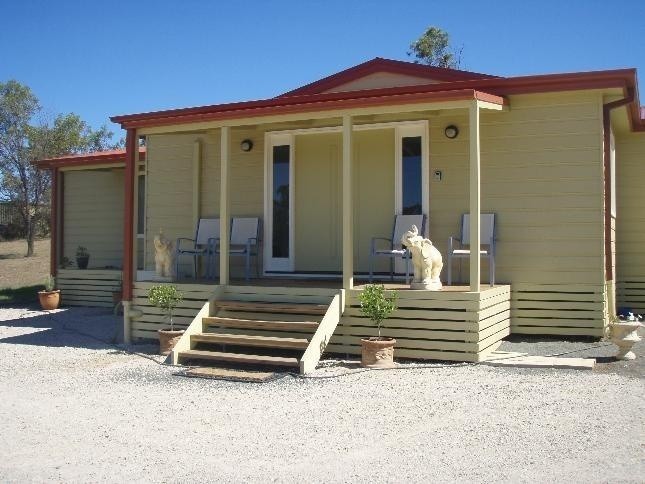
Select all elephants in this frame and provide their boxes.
[152,229,177,279]
[400,224,446,285]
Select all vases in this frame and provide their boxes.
[608,321,641,361]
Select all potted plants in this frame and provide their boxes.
[144,283,185,356]
[75,246,90,269]
[356,284,400,367]
[111,268,123,304]
[37,274,60,311]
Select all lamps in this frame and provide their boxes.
[444,124,459,139]
[240,139,252,151]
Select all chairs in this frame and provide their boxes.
[369,214,498,288]
[176,215,262,283]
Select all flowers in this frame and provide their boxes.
[607,311,642,323]
